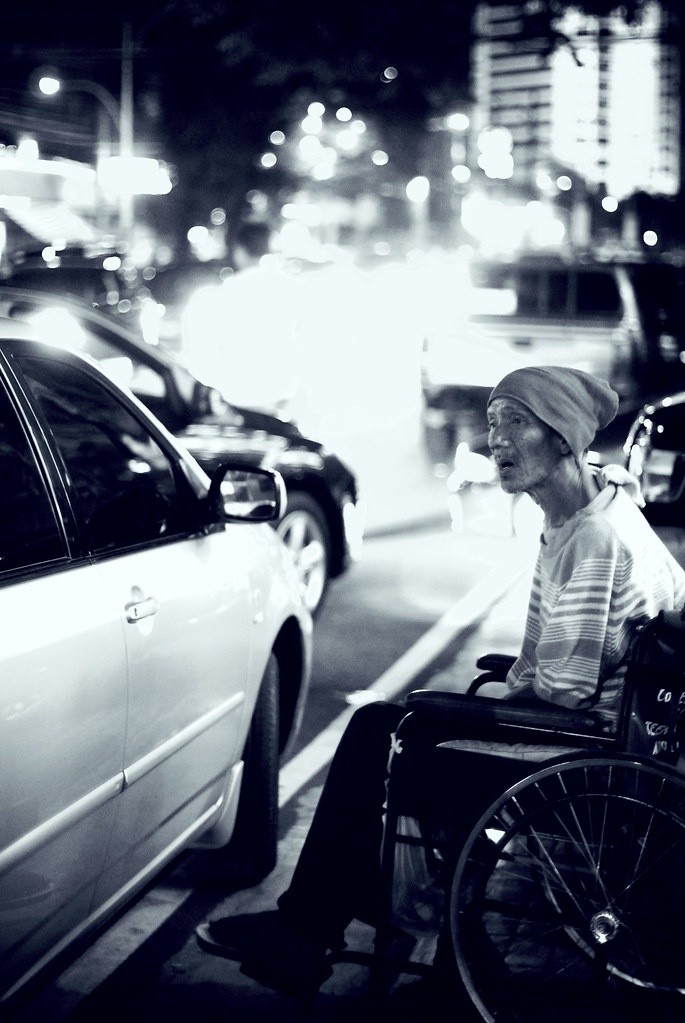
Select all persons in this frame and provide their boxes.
[195,364,685,995]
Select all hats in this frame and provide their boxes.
[487,365,619,458]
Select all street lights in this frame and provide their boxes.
[40,76,134,302]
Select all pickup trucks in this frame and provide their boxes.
[418,256,685,467]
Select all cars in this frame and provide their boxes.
[1,339,319,1014]
[0,288,361,623]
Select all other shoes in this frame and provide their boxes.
[207,907,346,974]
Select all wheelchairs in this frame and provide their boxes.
[242,612,685,1023]
